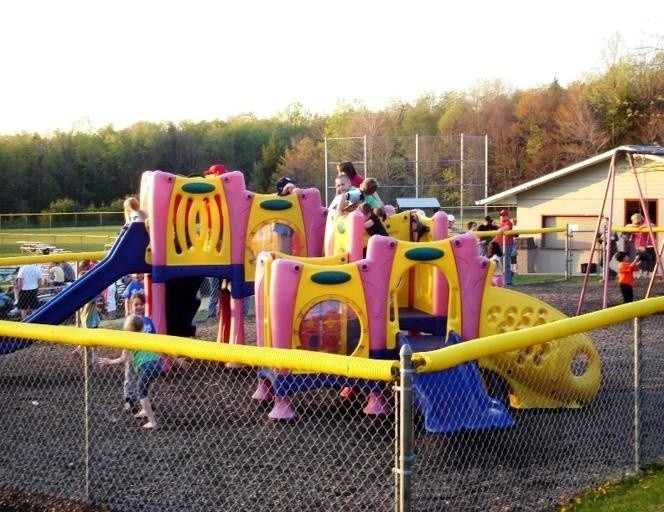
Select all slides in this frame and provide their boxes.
[1,222,149,355]
[396,330,517,433]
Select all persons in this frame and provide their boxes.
[447,215,456,232]
[96,315,191,428]
[615,251,643,303]
[329,161,390,237]
[597,217,619,283]
[620,224,635,256]
[122,292,156,410]
[631,213,655,278]
[274,176,304,255]
[466,209,515,287]
[0,259,144,328]
[432,208,438,216]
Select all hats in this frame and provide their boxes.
[484,216,493,221]
[615,251,629,260]
[203,164,229,175]
[631,213,644,224]
[447,214,455,222]
[500,210,509,215]
[275,176,296,192]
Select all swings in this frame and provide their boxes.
[634,156,656,272]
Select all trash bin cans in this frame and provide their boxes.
[516,238,538,273]
